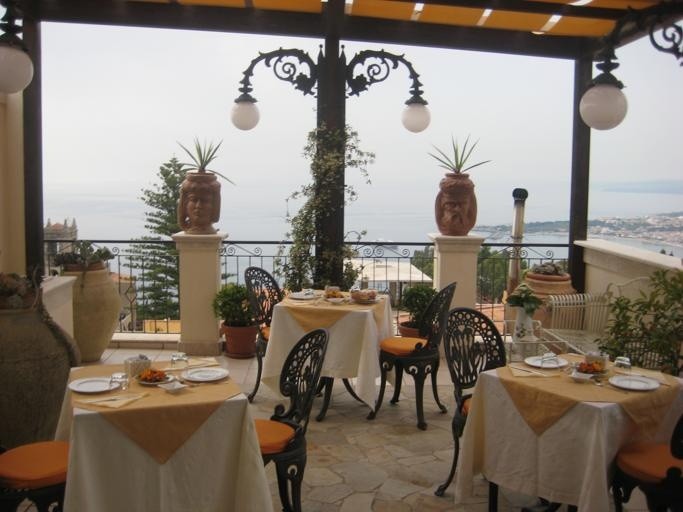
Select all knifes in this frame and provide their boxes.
[510,366,546,376]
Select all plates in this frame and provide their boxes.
[133,375,174,385]
[351,297,383,304]
[68,378,120,393]
[182,368,229,381]
[608,374,660,391]
[287,292,314,299]
[524,354,568,368]
[327,298,344,303]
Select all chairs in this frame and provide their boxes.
[366,281,457,431]
[434,308,506,512]
[253,329,329,512]
[0,441,69,512]
[244,267,326,404]
[607,415,683,512]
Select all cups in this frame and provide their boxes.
[606,357,632,394]
[106,371,128,399]
[170,352,189,385]
[541,351,560,375]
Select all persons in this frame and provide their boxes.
[178,172,220,234]
[433,173,476,236]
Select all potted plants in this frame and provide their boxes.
[55,239,123,362]
[427,134,491,236]
[505,262,578,355]
[399,284,441,339]
[506,283,542,360]
[212,282,262,358]
[174,137,236,234]
